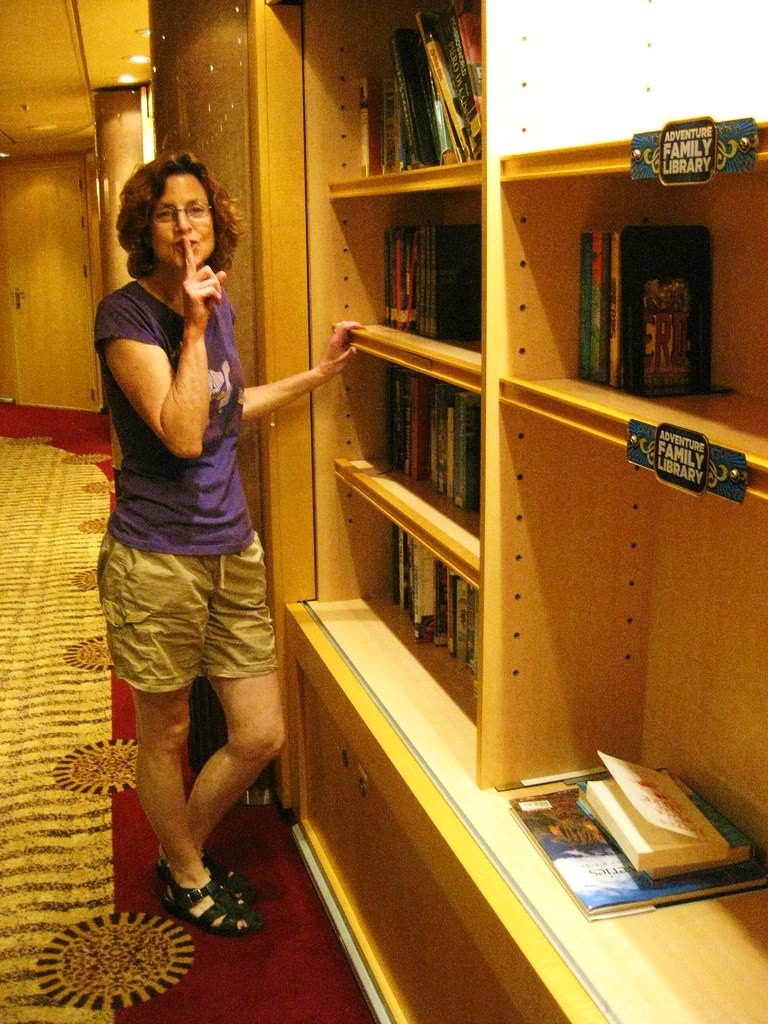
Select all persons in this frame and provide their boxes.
[93,151,368,937]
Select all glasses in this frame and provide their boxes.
[151,203,214,223]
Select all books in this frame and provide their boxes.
[507,749,768,921]
[390,524,479,699]
[381,365,483,511]
[382,223,482,343]
[579,221,717,394]
[358,0,483,177]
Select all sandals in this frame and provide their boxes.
[160,867,264,936]
[155,845,256,907]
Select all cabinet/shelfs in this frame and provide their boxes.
[150,1,768,1024]
